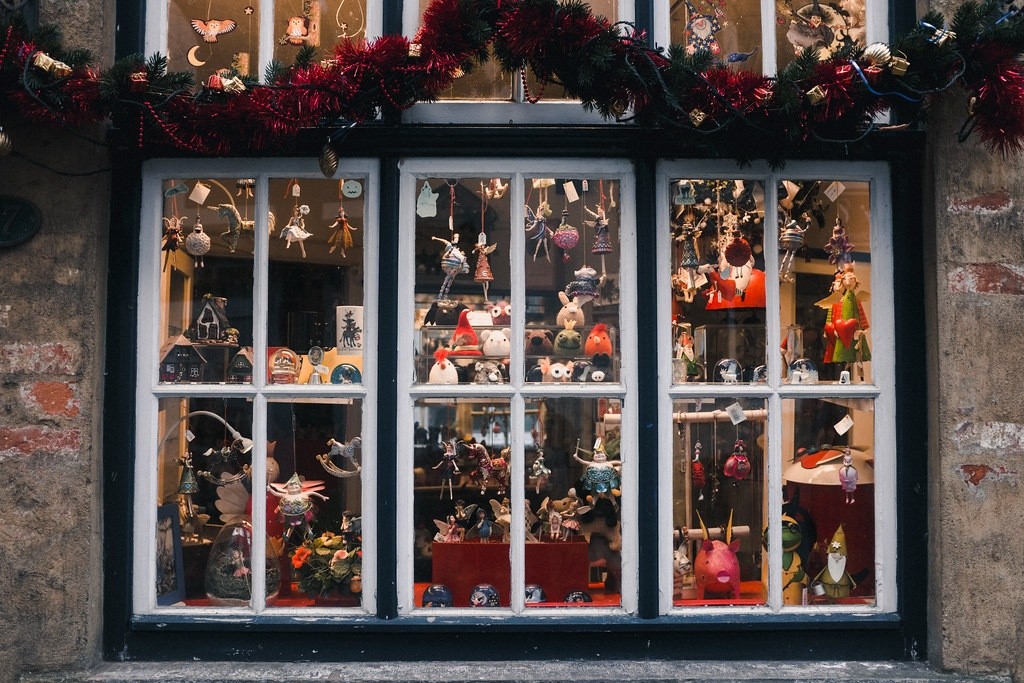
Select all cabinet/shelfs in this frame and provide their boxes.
[157,324,875,608]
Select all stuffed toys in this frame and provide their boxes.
[426,291,611,384]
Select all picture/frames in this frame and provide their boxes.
[158,504,187,607]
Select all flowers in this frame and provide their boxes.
[278,524,361,599]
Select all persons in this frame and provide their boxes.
[691,440,706,501]
[573,437,621,501]
[839,449,858,504]
[583,203,613,280]
[432,439,511,500]
[280,206,313,257]
[185,215,211,267]
[432,233,497,303]
[533,442,551,494]
[810,525,856,598]
[524,205,579,262]
[447,496,532,543]
[722,440,750,486]
[549,499,580,542]
[161,216,188,272]
[328,208,357,258]
[824,217,855,263]
[677,222,699,291]
[822,266,871,365]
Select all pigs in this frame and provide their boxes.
[694,539,740,600]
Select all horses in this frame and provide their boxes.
[323,437,361,471]
[469,444,512,495]
[201,438,245,475]
[217,204,275,254]
